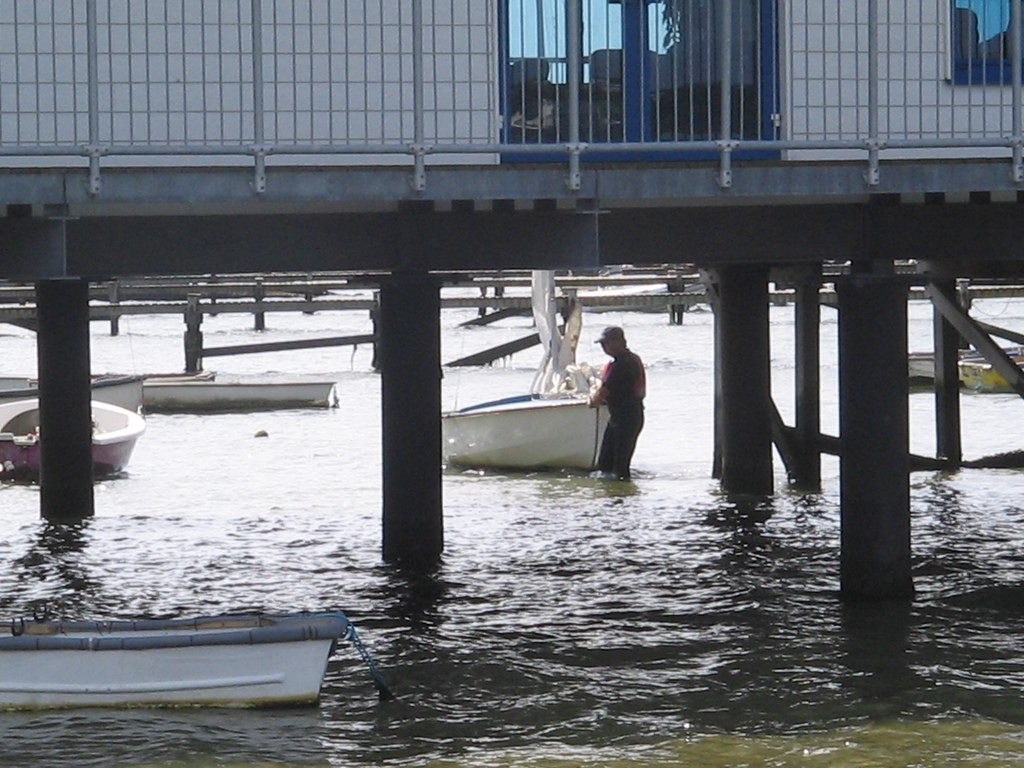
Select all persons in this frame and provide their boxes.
[664,275,685,325]
[587,326,646,479]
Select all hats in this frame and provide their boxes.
[593,326,624,343]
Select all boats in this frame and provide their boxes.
[0,609,352,714]
[0,365,339,486]
[438,394,611,474]
[908,344,1024,394]
